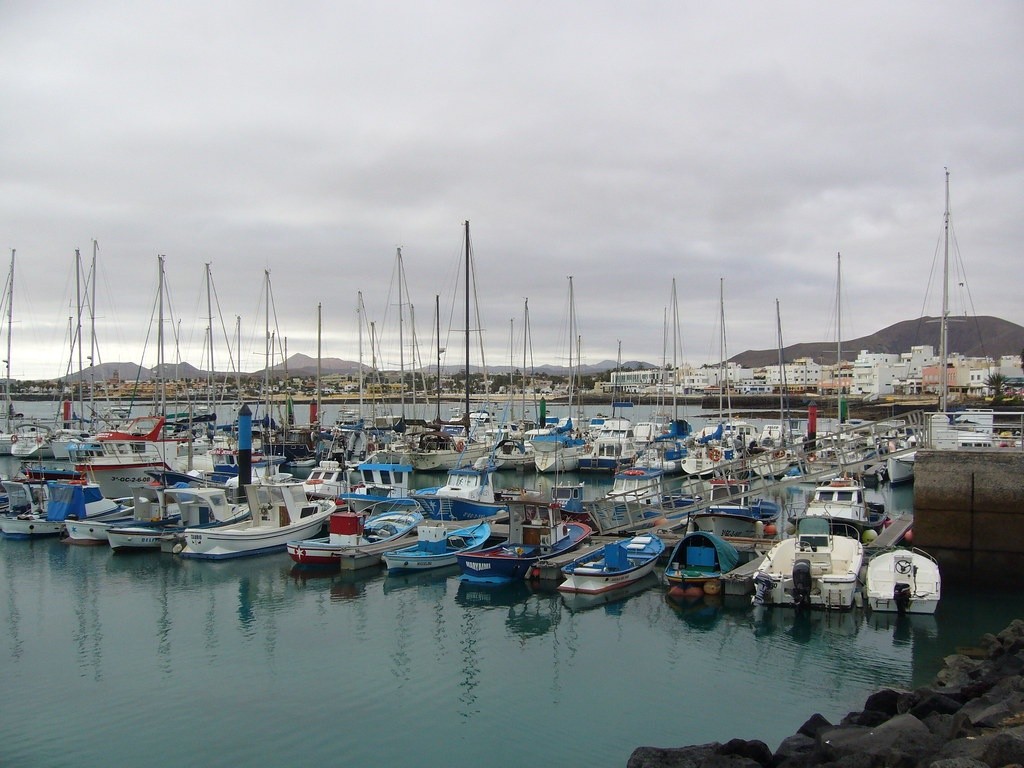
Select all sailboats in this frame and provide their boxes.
[1,168,1023,484]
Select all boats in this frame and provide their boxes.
[0,478,250,553]
[455,501,593,584]
[785,476,887,544]
[662,532,740,597]
[66,416,262,500]
[379,522,491,573]
[865,546,943,614]
[687,475,780,539]
[750,515,865,608]
[286,509,423,566]
[180,484,338,561]
[556,531,666,594]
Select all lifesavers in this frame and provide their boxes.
[70,479,88,485]
[709,449,722,461]
[624,469,645,476]
[227,438,235,446]
[807,453,816,463]
[366,443,375,453]
[10,435,18,443]
[457,440,464,453]
[306,479,324,485]
